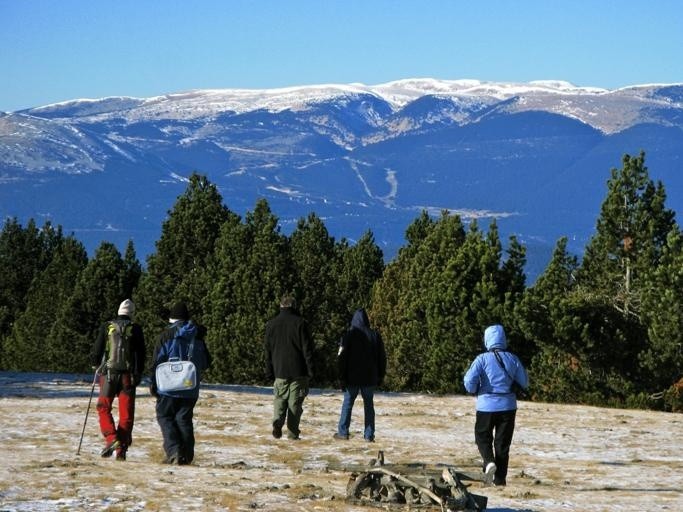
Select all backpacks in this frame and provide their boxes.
[100,321,136,386]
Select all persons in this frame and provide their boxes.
[264,297,314,442]
[462,322,528,486]
[149,301,214,469]
[333,309,388,442]
[87,297,146,465]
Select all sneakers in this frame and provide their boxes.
[333,433,349,440]
[100,437,126,461]
[483,461,497,482]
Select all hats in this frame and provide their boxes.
[169,301,188,324]
[118,298,136,319]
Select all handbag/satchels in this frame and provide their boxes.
[511,381,530,400]
[155,357,196,392]
